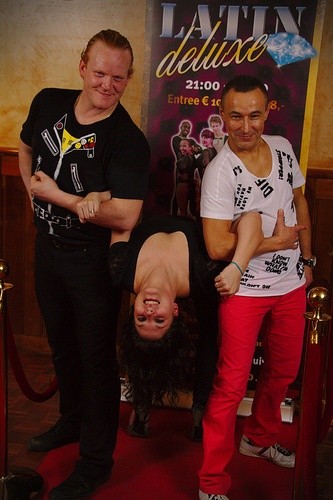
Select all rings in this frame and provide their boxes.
[89,211,93,213]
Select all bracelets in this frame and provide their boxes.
[230,262,243,276]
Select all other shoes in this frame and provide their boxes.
[47,457,113,500]
[29,423,80,452]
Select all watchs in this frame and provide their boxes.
[303,256,316,267]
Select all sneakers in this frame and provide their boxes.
[238,431,295,469]
[198,488,230,500]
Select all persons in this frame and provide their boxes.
[199,74,314,500]
[76,190,264,442]
[172,115,228,221]
[18,29,151,500]
